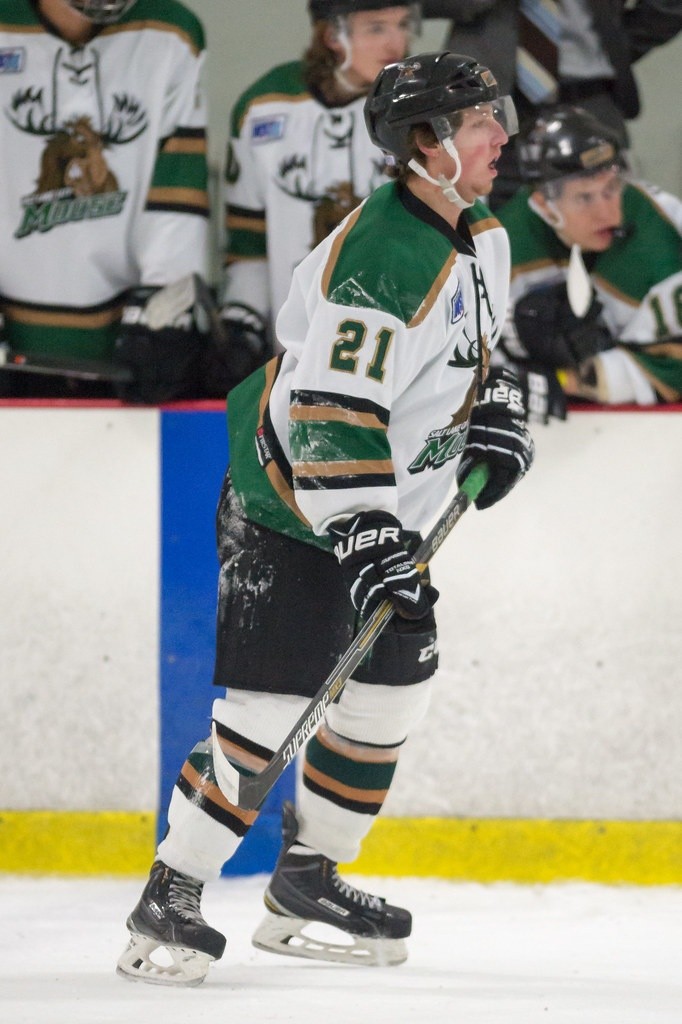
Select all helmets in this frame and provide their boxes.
[305,2,408,22]
[516,97,630,183]
[363,51,500,165]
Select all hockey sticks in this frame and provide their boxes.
[209,460,492,812]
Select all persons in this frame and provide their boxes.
[207,0,425,397]
[424,0,681,426]
[111,52,537,986]
[0,0,217,404]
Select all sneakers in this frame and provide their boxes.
[251,839,411,968]
[114,858,226,988]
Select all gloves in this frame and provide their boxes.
[330,509,439,636]
[455,365,536,509]
[116,275,275,406]
